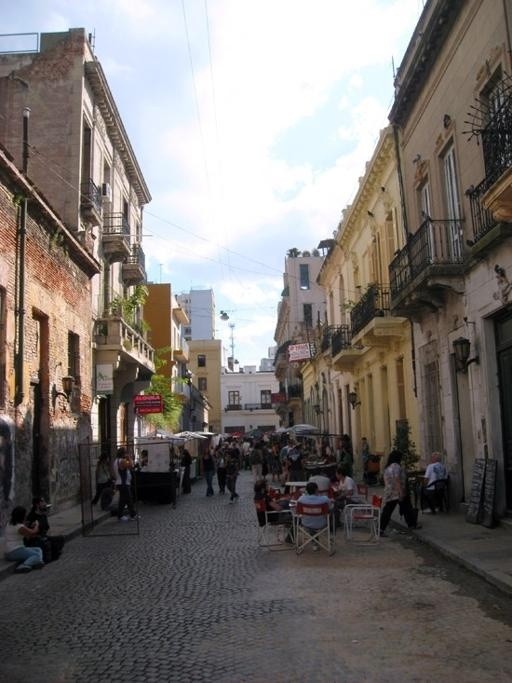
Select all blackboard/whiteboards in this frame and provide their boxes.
[467,459,496,527]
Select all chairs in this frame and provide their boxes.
[253,474,383,556]
[413,477,448,515]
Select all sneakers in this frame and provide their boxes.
[120,513,141,522]
[410,524,423,530]
[14,566,32,574]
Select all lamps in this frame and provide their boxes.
[314,404,323,416]
[452,336,479,375]
[348,392,361,409]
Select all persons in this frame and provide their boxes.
[23,496,65,563]
[4,506,43,573]
[376,451,422,537]
[415,453,448,514]
[92,448,147,521]
[180,424,369,551]
